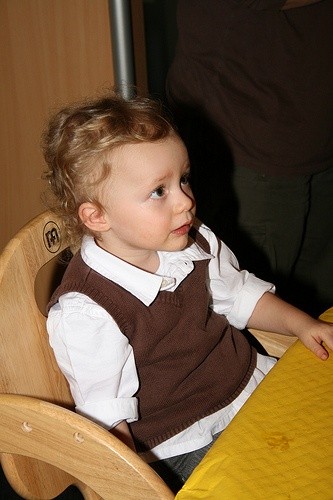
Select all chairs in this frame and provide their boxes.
[0,207,299,500]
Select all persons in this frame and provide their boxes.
[41,85,333,495]
[164,1,333,320]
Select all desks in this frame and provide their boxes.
[172,304,333,500]
